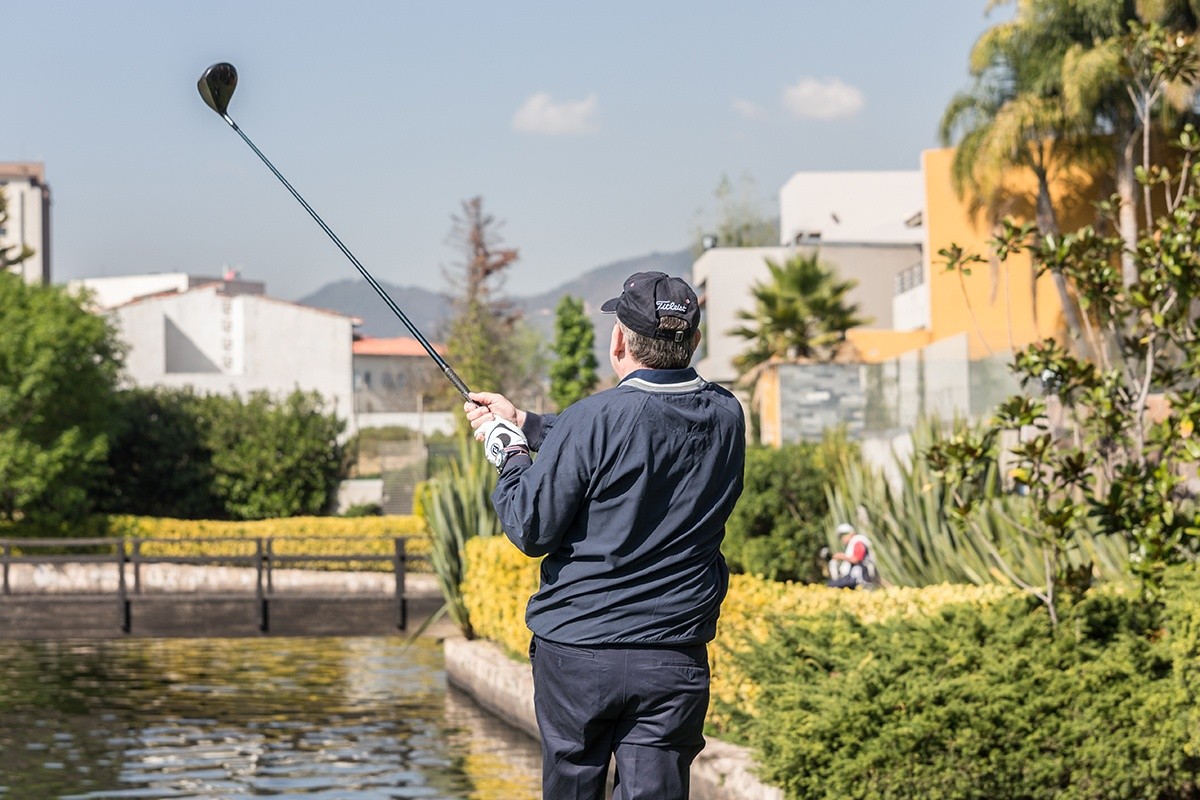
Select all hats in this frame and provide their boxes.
[836,523,853,536]
[601,271,701,342]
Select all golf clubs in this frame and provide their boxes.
[196,61,471,403]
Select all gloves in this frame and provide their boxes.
[474,414,528,463]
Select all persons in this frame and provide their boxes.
[464,273,745,800]
[829,522,879,589]
